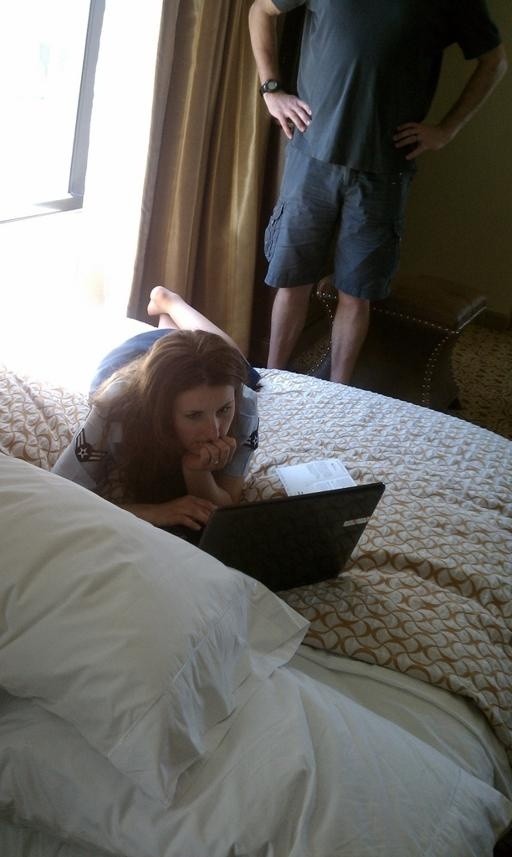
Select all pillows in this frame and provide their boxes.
[1,457,313,807]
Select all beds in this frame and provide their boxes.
[0,306,511,857]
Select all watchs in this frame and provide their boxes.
[260,78,283,97]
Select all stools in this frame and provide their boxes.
[306,272,489,416]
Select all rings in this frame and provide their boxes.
[210,460,220,465]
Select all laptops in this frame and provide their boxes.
[158,481,386,592]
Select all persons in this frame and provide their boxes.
[244,0,509,385]
[49,285,261,532]
